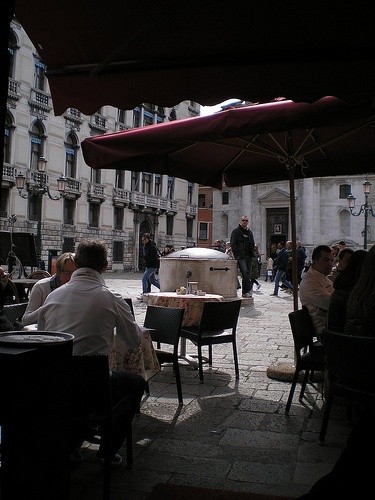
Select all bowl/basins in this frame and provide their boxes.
[198,292,205,295]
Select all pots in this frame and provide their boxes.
[158,247,238,298]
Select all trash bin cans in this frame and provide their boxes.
[47,249,61,275]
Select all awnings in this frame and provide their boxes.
[12,0,375,116]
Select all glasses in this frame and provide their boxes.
[242,220,248,221]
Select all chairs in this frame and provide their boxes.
[143,305,188,406]
[26,270,51,279]
[285,304,326,411]
[70,353,150,500]
[180,299,242,381]
[3,302,38,325]
[318,327,375,442]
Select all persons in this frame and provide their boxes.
[264,241,307,297]
[300,241,375,372]
[213,216,262,298]
[36,238,137,465]
[136,233,176,301]
[22,252,80,331]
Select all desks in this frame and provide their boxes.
[11,278,40,301]
[142,291,226,370]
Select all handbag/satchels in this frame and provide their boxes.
[268,271,272,276]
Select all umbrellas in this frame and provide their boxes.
[81,96,375,312]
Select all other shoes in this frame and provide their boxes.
[279,285,291,294]
[136,294,143,300]
[256,284,261,291]
[95,450,123,467]
[270,292,278,296]
[242,293,252,298]
